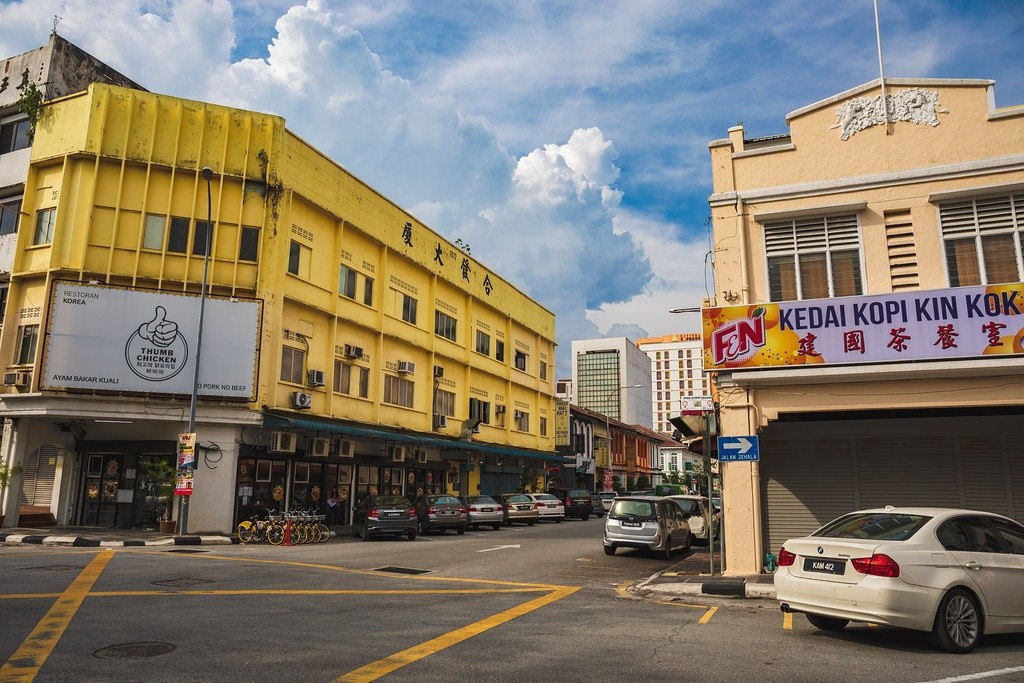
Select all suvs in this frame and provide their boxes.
[544,488,593,521]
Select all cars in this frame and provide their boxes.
[458,496,504,532]
[492,494,539,525]
[689,490,720,496]
[414,494,468,535]
[667,494,720,542]
[602,496,692,558]
[591,491,618,517]
[774,502,1024,653]
[526,494,566,524]
[352,495,418,541]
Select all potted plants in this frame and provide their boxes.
[139,458,179,533]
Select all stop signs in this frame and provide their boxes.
[693,480,697,484]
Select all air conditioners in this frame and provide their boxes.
[434,413,447,428]
[289,391,312,409]
[3,372,29,386]
[308,370,325,386]
[398,361,415,374]
[473,424,481,433]
[388,446,405,461]
[514,410,522,419]
[343,343,364,358]
[434,365,444,377]
[414,449,428,463]
[270,431,355,457]
[495,405,505,414]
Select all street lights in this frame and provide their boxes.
[178,167,214,537]
[606,384,642,492]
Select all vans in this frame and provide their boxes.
[654,484,689,496]
[623,491,646,495]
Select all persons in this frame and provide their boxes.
[327,487,344,526]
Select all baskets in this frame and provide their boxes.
[264,515,281,521]
[284,515,326,522]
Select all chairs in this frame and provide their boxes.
[622,504,647,518]
[964,525,994,551]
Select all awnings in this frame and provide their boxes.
[262,413,573,464]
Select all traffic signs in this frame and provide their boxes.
[718,436,759,461]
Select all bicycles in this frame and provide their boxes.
[238,507,330,546]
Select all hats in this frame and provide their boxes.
[417,488,423,494]
